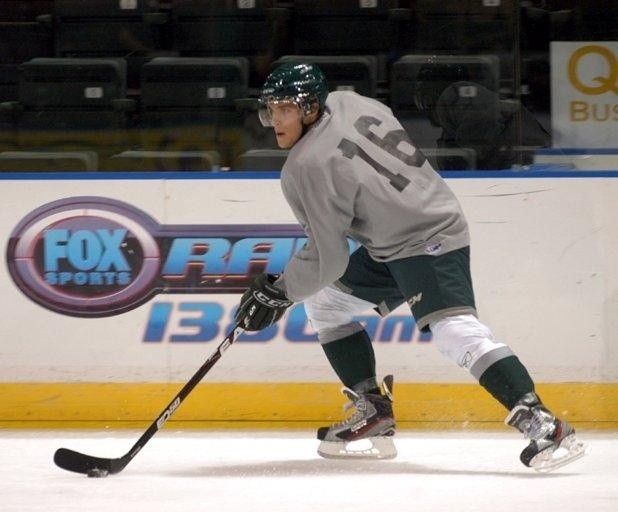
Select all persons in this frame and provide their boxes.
[435,80,552,167]
[232,62,589,473]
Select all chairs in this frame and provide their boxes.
[0,0,590,170]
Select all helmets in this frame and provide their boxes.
[258,62,326,127]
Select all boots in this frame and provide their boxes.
[504,391,574,466]
[317,373,396,442]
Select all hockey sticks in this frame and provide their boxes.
[55,299,262,474]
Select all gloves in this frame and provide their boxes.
[236,273,291,335]
